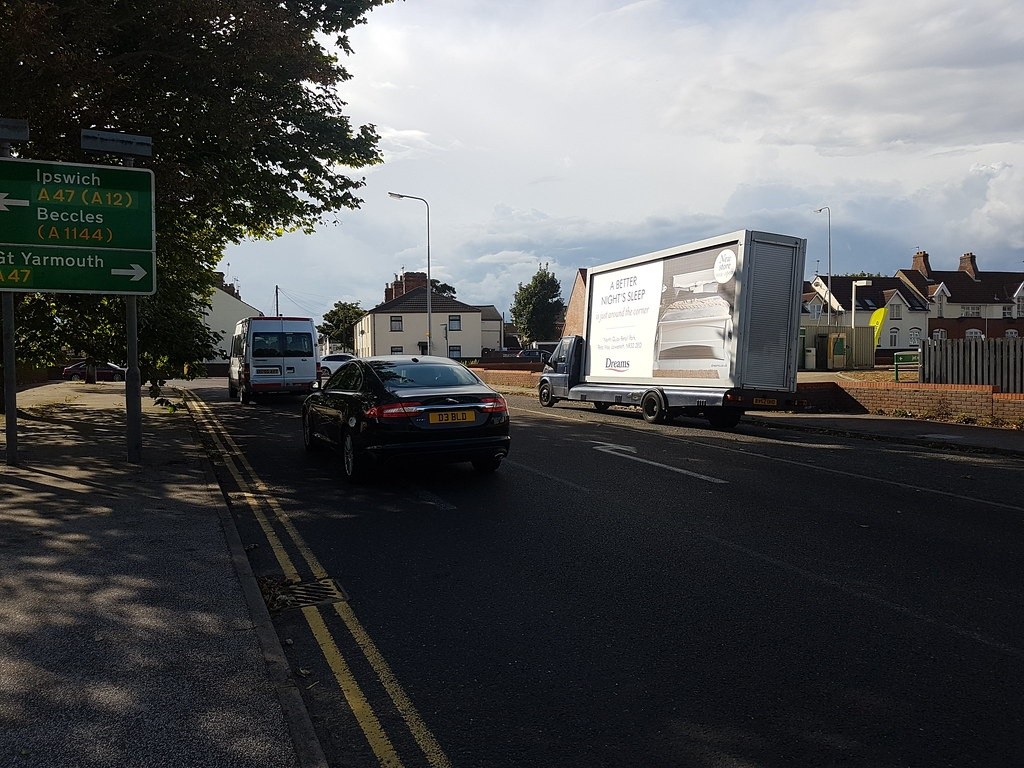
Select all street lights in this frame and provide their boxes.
[850,278,872,371]
[816,206,832,336]
[439,323,448,358]
[388,191,433,356]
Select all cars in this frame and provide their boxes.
[321,354,358,377]
[517,349,552,365]
[62,359,126,382]
[301,355,512,487]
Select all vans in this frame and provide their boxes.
[221,314,323,405]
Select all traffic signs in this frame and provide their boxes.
[0,157,158,296]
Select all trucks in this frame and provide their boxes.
[537,334,786,431]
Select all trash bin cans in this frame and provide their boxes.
[805,347,816,370]
